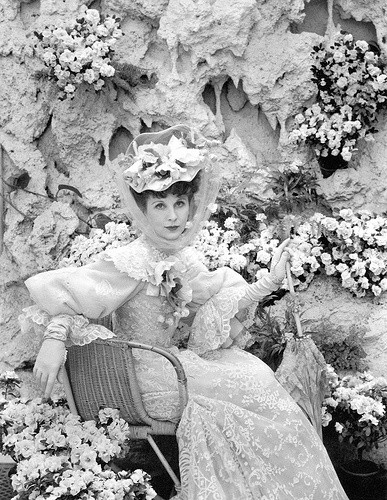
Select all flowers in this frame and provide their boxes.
[0,4,387,500]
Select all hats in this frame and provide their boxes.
[123,134,205,193]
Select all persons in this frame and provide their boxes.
[24,124,348,500]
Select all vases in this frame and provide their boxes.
[338,458,380,498]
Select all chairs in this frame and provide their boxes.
[60,310,315,500]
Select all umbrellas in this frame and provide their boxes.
[271,262,327,441]
